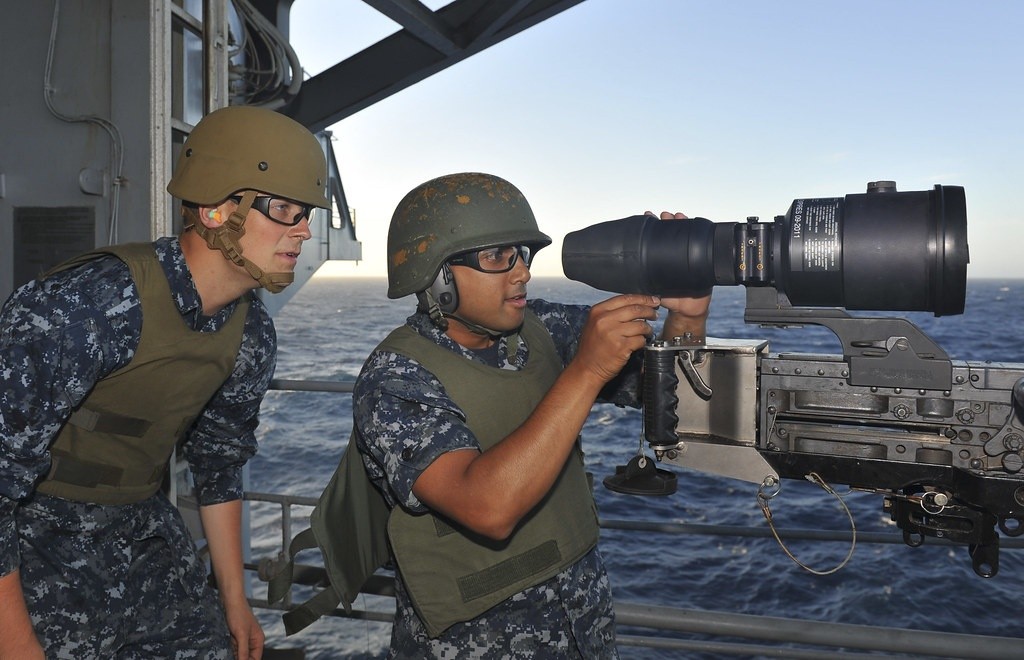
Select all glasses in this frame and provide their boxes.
[448,244,535,273]
[229,195,316,226]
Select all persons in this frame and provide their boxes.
[0,106,332,660]
[352,172,714,659]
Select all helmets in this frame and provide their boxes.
[167,104,333,211]
[386,171,552,298]
